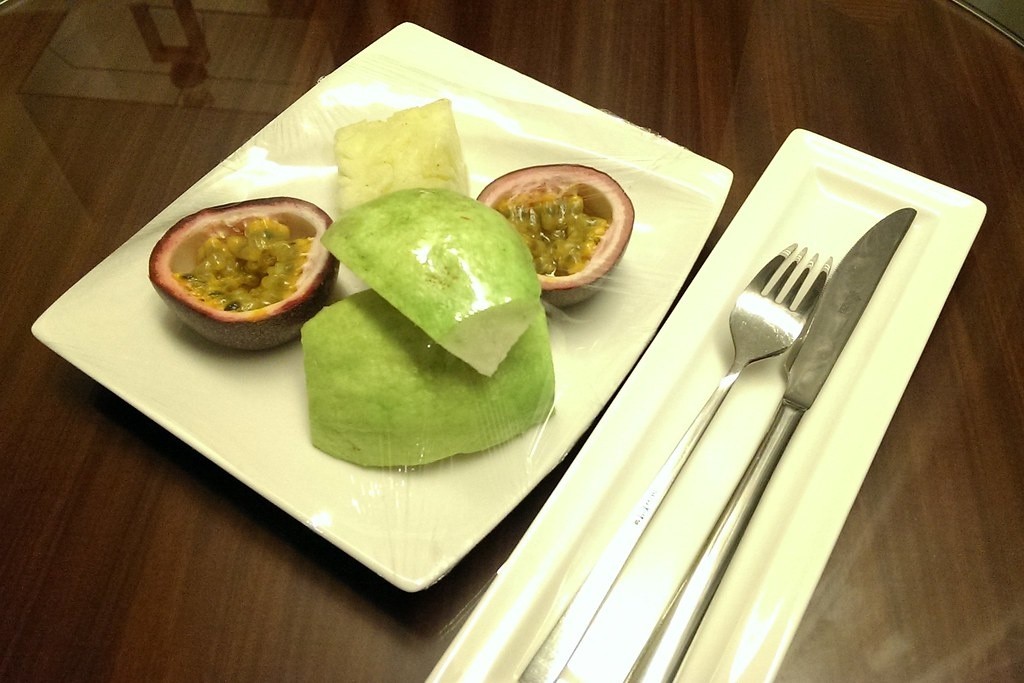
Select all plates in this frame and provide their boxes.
[29,21,735,592]
[425,124,988,681]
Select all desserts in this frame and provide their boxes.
[333,98,468,211]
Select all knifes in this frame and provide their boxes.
[621,207,919,683]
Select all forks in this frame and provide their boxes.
[516,244,834,683]
